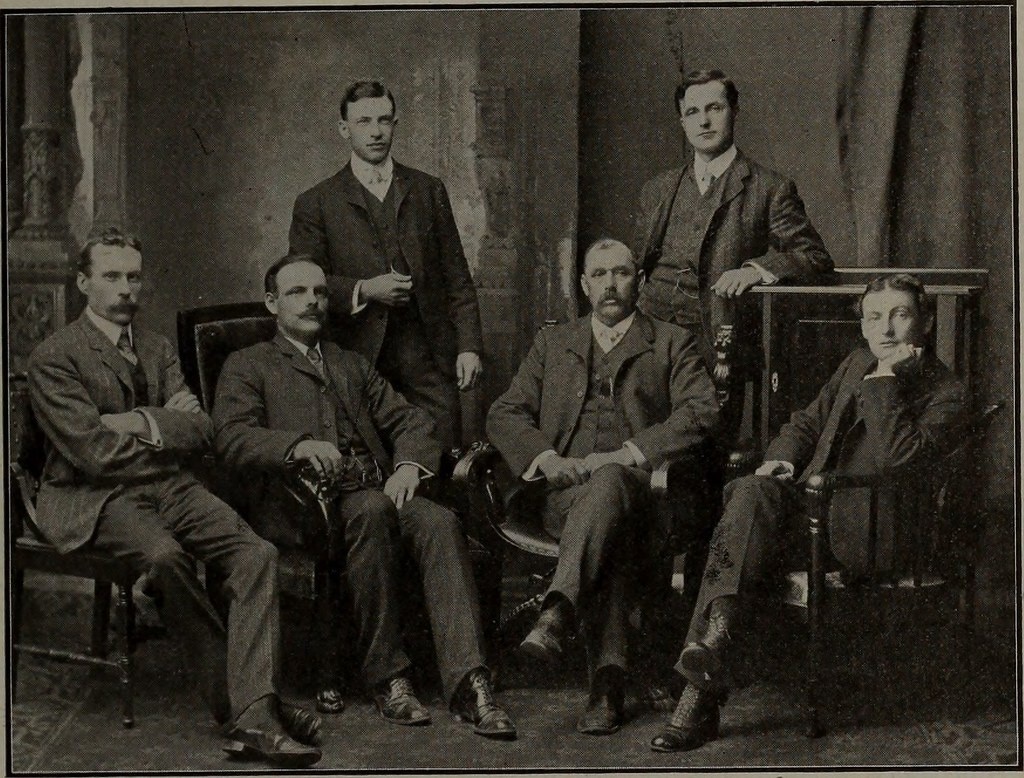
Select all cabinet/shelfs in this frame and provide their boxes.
[749,269,988,451]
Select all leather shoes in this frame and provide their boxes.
[453,668,515,734]
[275,697,322,747]
[682,612,731,680]
[371,678,431,725]
[651,683,720,753]
[221,717,322,767]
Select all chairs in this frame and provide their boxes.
[9,372,162,728]
[725,397,1005,740]
[176,306,503,690]
[457,318,738,699]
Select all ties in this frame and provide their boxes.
[702,167,714,192]
[371,167,386,202]
[606,329,618,353]
[117,327,137,365]
[306,348,324,378]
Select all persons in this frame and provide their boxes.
[486,239,724,735]
[29,228,331,769]
[291,81,484,530]
[648,275,967,753]
[212,252,515,739]
[621,70,835,466]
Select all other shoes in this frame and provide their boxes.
[576,674,624,733]
[519,610,564,658]
